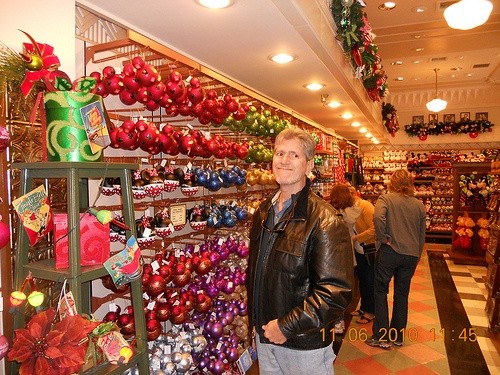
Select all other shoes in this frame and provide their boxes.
[393,341,404,347]
[364,338,392,350]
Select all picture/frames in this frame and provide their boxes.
[475,113,488,121]
[460,112,470,120]
[444,114,455,122]
[412,116,424,123]
[429,114,438,123]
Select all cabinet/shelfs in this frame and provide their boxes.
[358,148,492,266]
[6,162,150,375]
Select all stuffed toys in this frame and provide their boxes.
[413,174,453,213]
[404,150,456,177]
[362,150,408,172]
[458,174,493,208]
[358,171,392,205]
[455,148,500,163]
[451,215,489,252]
[425,214,454,232]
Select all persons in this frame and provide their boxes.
[328,184,378,324]
[244,129,358,375]
[364,169,427,349]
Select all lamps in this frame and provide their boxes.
[426,69,447,112]
[444,0,491,30]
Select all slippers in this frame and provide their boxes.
[351,310,364,316]
[356,316,376,324]
